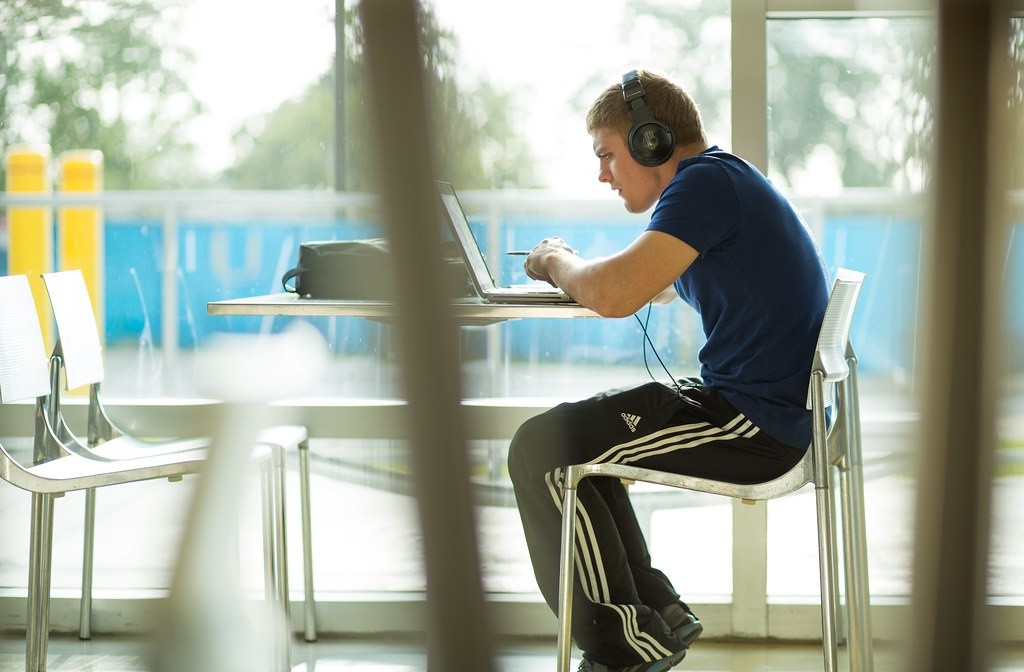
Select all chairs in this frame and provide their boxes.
[0,274,320,672]
[557,264,874,672]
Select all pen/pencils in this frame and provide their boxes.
[505,250,580,255]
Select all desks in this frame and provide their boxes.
[206,296,610,672]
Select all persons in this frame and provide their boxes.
[508,69,832,672]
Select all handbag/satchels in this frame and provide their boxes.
[281,239,495,300]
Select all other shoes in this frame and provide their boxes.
[577,649,686,672]
[671,614,704,646]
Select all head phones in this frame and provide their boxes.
[622,70,677,167]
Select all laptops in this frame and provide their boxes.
[435,179,580,304]
